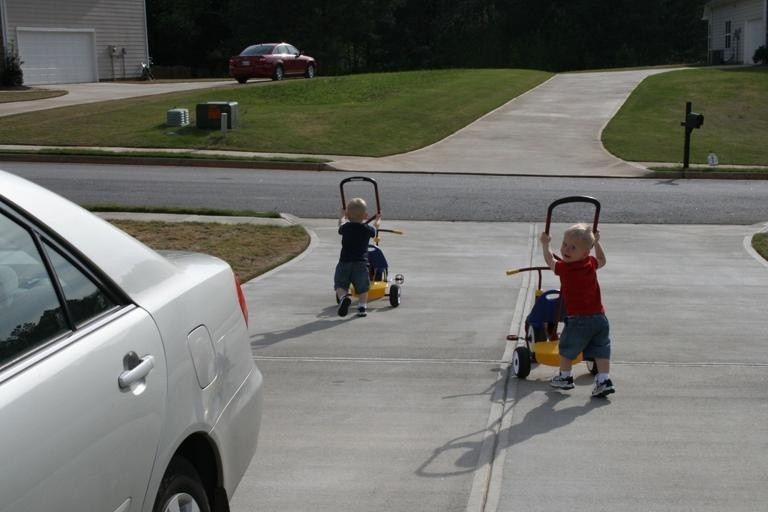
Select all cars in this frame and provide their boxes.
[0,171,265,511]
[229,42,317,84]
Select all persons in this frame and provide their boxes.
[334,197,382,317]
[540,223,616,398]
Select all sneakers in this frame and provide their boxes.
[357,305,368,317]
[337,296,352,318]
[547,373,575,390]
[590,379,616,398]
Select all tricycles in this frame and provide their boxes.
[504,195,602,378]
[333,174,405,310]
